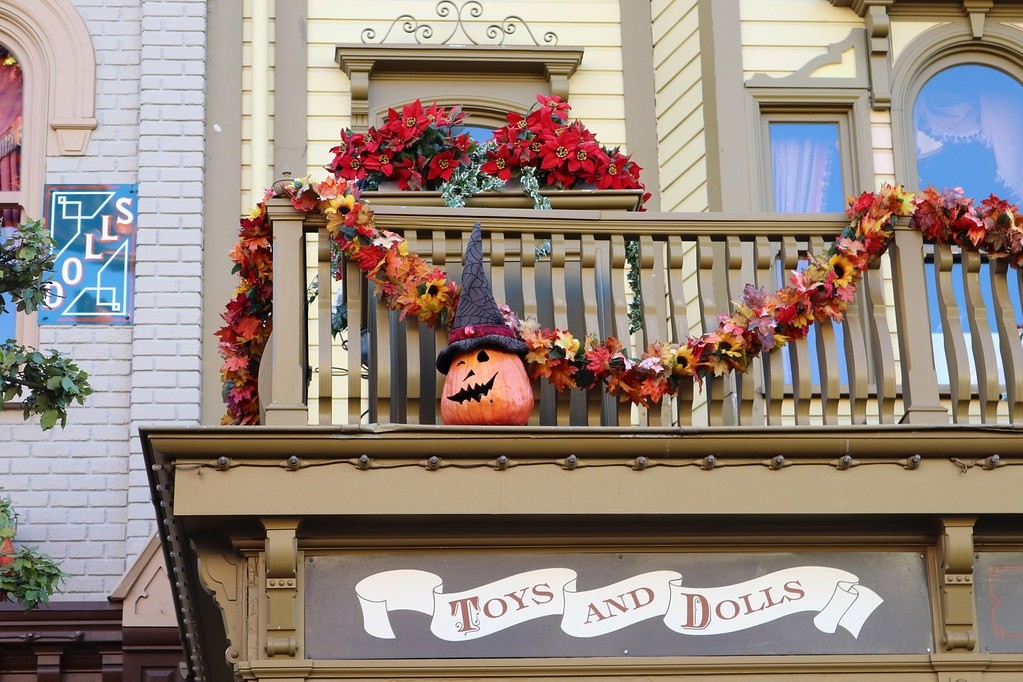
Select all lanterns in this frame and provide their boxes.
[435,221,534,425]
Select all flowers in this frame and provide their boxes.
[323,93,652,211]
